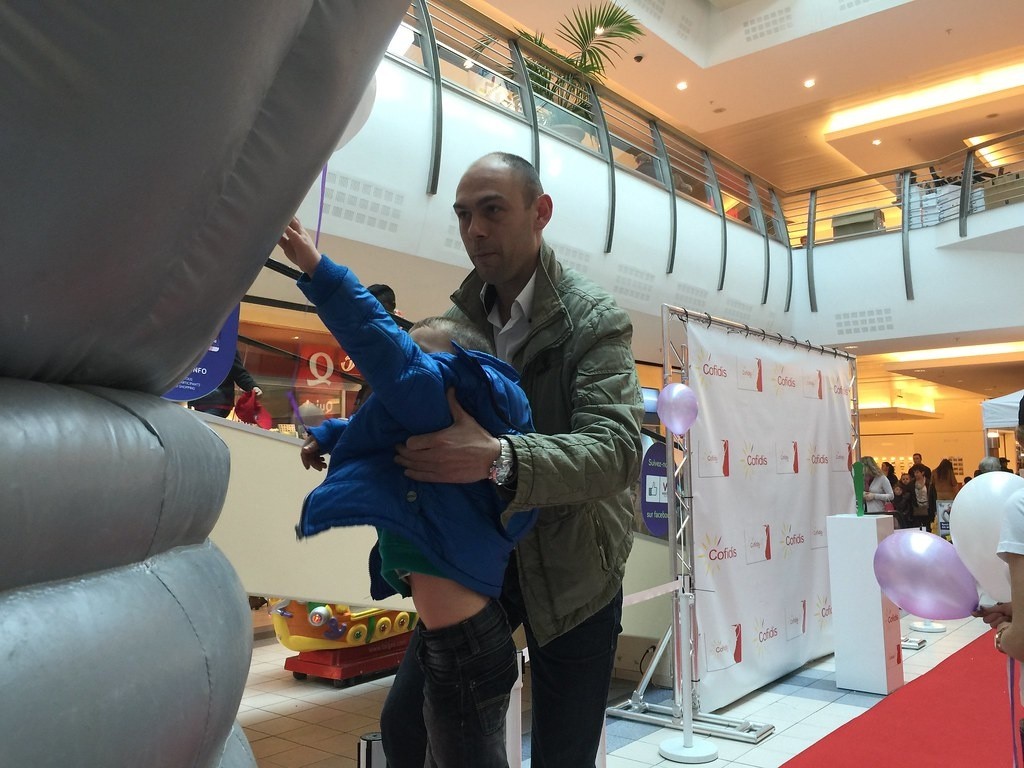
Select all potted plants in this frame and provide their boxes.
[465,0,646,143]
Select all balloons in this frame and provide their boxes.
[874,529,982,620]
[950,470,1024,603]
[656,383,699,439]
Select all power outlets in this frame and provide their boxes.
[649,642,657,656]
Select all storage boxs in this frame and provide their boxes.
[984,171,1024,212]
[832,208,886,240]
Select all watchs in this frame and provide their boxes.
[488,438,519,486]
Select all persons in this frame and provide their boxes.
[367,284,397,317]
[861,456,894,513]
[188,347,263,418]
[999,458,1013,474]
[971,395,1024,768]
[931,459,957,500]
[276,213,540,768]
[882,453,983,533]
[978,456,1001,472]
[395,151,646,768]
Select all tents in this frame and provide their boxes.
[981,386,1024,474]
